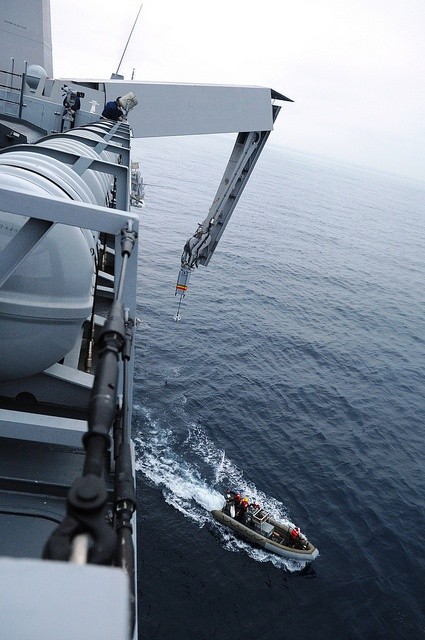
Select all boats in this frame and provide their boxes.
[211,498,319,561]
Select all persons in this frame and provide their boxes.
[251,500,260,510]
[289,527,301,545]
[241,497,249,512]
[99,96,123,121]
[227,489,241,514]
[61,96,81,133]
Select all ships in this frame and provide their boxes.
[1,0,296,640]
